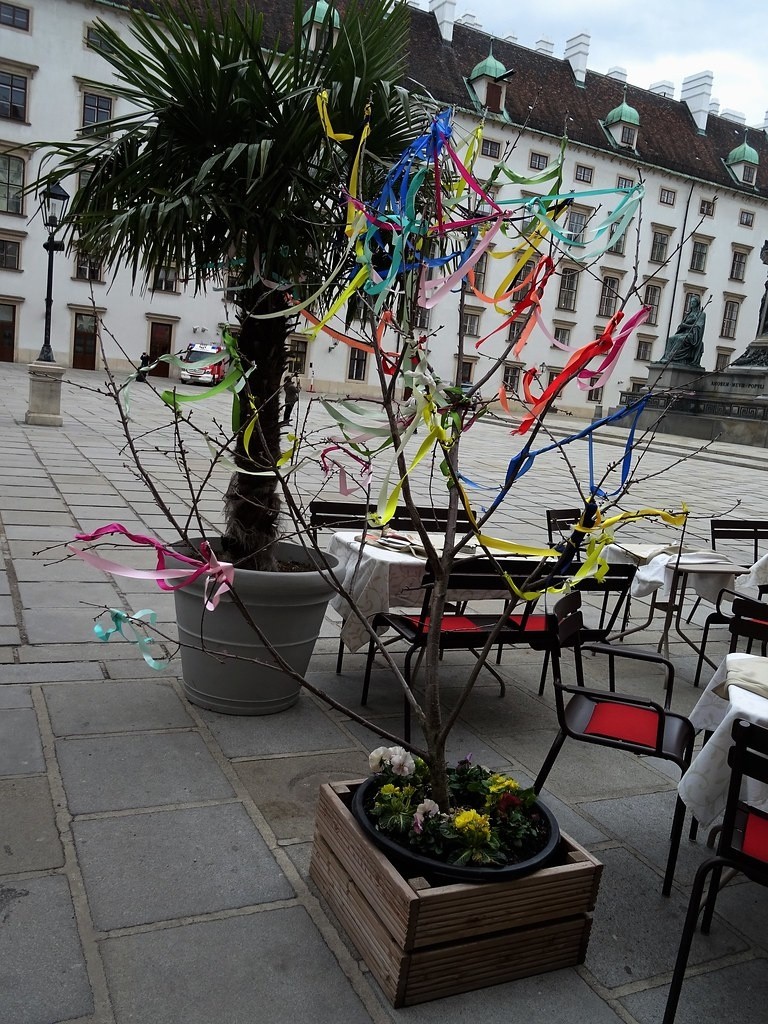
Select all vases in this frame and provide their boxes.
[351,764,559,882]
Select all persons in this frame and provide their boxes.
[136,351,151,382]
[660,296,706,367]
[284,371,302,425]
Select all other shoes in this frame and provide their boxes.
[288,419,293,420]
[284,421,293,426]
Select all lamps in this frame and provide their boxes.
[536,362,547,378]
[329,335,340,353]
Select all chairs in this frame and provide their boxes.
[543,508,768,1024]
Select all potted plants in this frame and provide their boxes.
[32,80,741,1009]
[0,0,475,717]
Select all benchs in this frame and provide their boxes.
[306,502,637,707]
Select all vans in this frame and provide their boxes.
[444,381,477,411]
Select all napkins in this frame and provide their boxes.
[646,541,728,565]
[711,658,768,701]
[354,525,444,559]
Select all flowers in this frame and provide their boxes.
[369,746,544,866]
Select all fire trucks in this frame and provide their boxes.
[179,342,228,388]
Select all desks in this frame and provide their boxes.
[596,545,751,653]
[326,524,559,707]
[670,653,768,937]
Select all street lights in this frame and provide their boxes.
[34,176,71,364]
